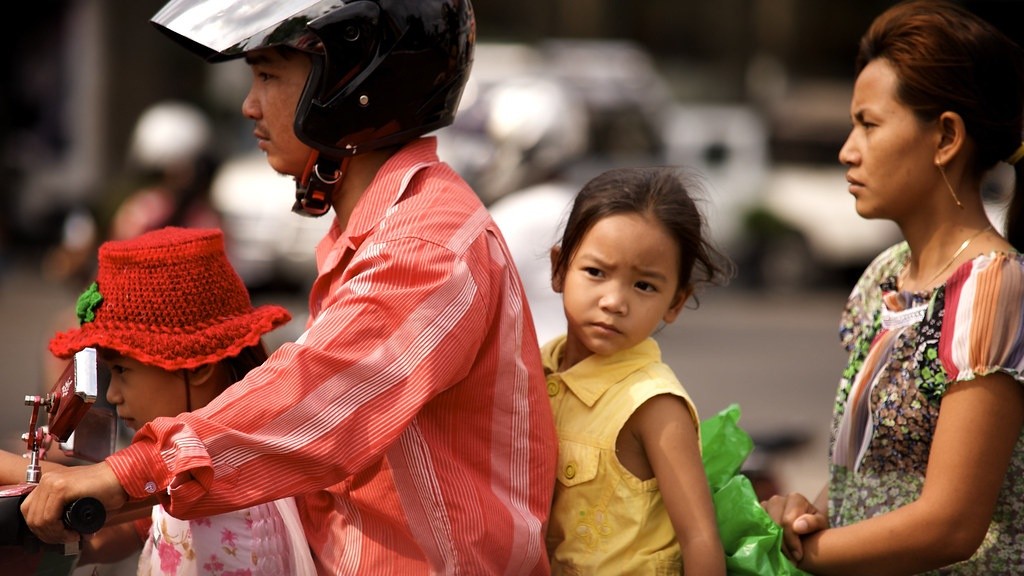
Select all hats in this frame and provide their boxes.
[47,227,293,371]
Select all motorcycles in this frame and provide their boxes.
[0,343,133,575]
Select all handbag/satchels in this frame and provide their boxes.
[701,403,813,576]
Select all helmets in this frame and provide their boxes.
[133,103,209,169]
[216,0,477,157]
[485,81,592,174]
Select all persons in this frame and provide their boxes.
[1,0,555,576]
[542,160,726,576]
[758,0,1024,576]
[1,222,318,576]
